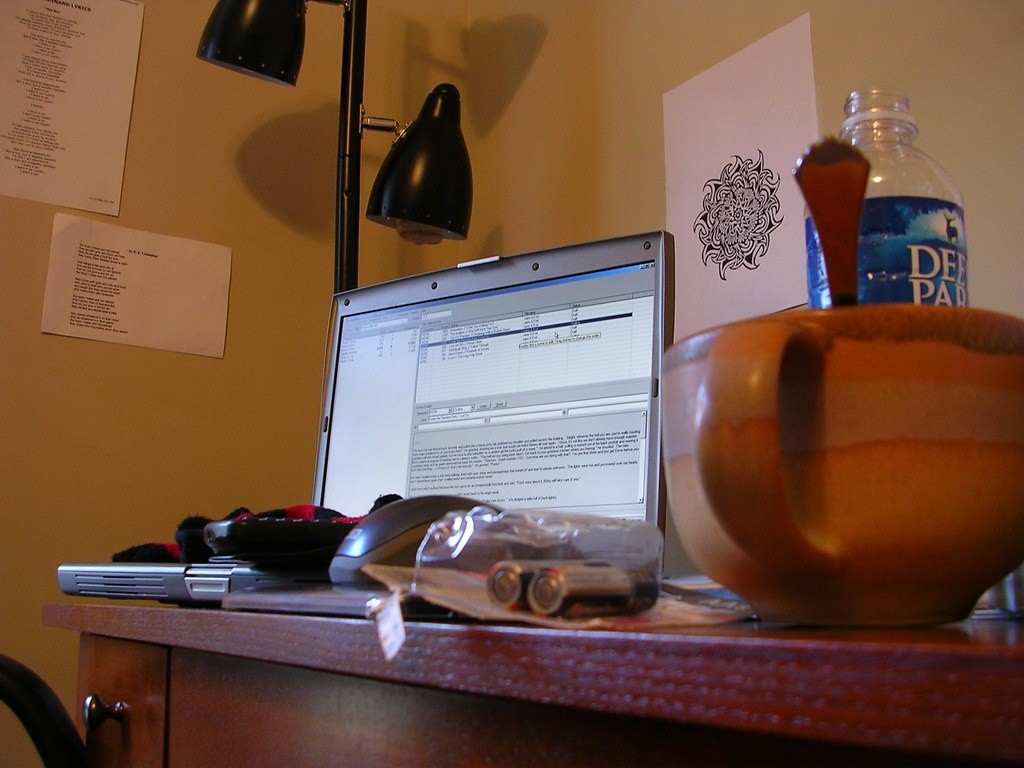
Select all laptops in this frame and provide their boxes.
[57,230,674,610]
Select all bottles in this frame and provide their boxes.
[805,87,967,306]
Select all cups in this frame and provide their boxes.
[662,303,1024,626]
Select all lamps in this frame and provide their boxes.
[196,0,352,87]
[359,82,473,246]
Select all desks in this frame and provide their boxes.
[39,602,1024,768]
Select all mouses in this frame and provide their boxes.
[327,491,497,588]
